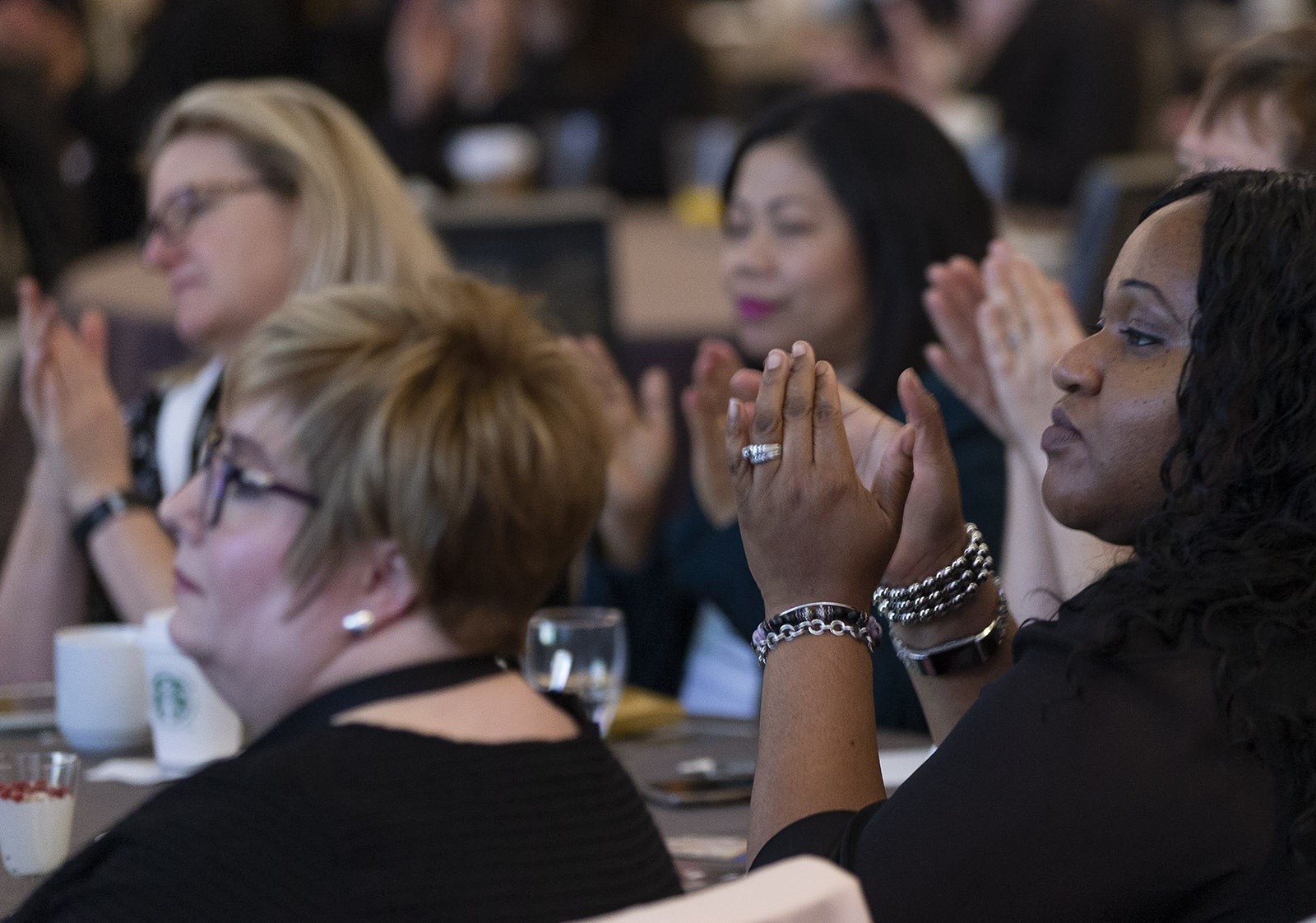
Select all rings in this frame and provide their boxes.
[742,441,782,465]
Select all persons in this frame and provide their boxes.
[0,1,1312,922]
[723,166,1315,922]
[1,276,682,922]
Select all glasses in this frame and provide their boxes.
[196,439,324,528]
[139,181,278,245]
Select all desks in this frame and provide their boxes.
[0,674,929,923]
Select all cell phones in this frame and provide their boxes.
[645,767,758,807]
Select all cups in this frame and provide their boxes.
[51,619,154,748]
[144,605,243,768]
[521,604,626,743]
[0,750,81,875]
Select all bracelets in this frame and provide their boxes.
[877,523,983,600]
[876,544,988,612]
[74,491,151,540]
[751,601,882,645]
[757,619,875,663]
[878,557,993,626]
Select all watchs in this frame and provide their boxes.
[888,578,1009,678]
